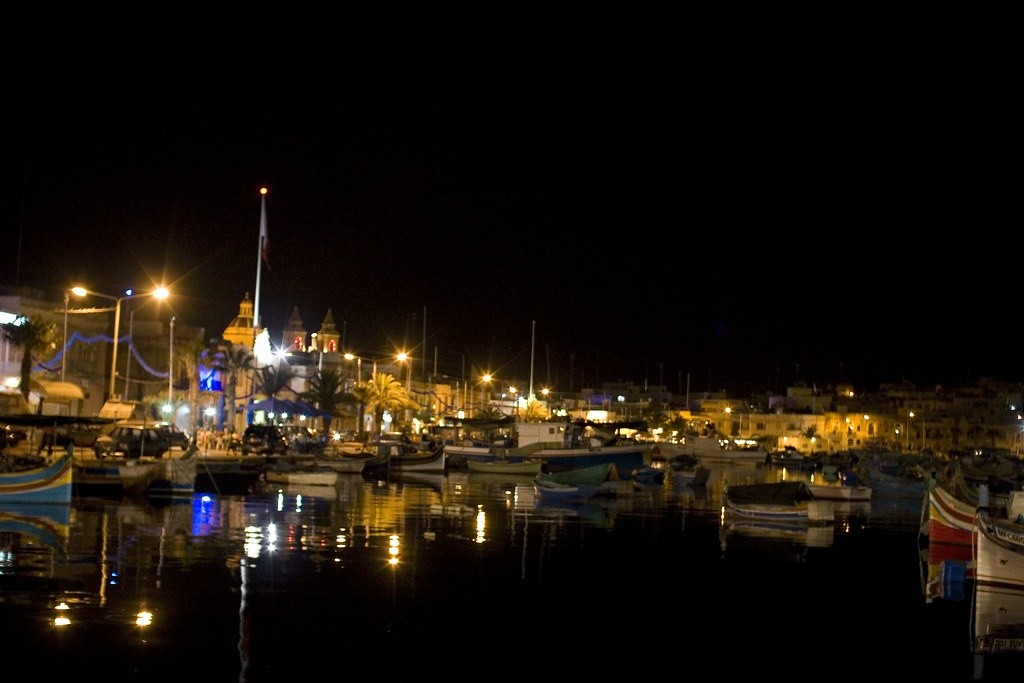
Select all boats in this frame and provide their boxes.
[391,471,445,496]
[719,504,834,550]
[1,418,1023,591]
[0,502,71,564]
[264,483,338,501]
[808,499,871,516]
[972,591,1023,678]
[923,542,982,610]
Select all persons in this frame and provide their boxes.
[187,425,314,456]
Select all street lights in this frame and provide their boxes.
[70,283,170,400]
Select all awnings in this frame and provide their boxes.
[30,380,84,402]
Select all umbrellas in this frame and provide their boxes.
[216,392,226,432]
[239,397,330,420]
